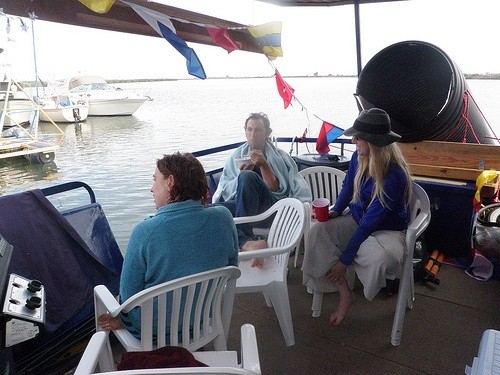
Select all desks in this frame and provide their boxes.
[412,174,479,270]
[292,152,351,171]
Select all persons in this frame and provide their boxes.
[213,111,313,270]
[311,108,412,326]
[98,151,238,347]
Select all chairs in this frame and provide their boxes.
[311,183,433,346]
[74,322,262,375]
[233,196,306,348]
[293,166,347,268]
[94,266,242,353]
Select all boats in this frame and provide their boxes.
[46,75,154,116]
[0,18,90,164]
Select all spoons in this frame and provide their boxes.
[244,153,249,157]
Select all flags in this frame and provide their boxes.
[316,121,345,155]
[249,22,284,60]
[131,6,206,79]
[205,24,242,54]
[275,70,294,108]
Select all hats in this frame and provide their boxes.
[344,108,402,146]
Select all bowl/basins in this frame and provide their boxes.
[236,157,252,167]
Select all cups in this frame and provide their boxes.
[312,197,330,222]
